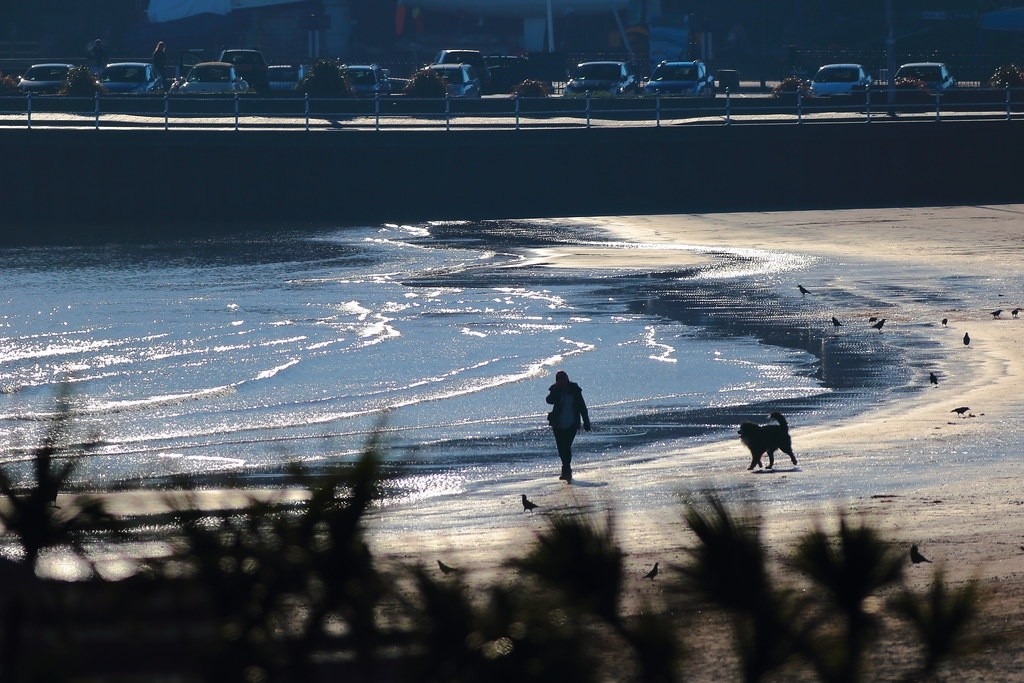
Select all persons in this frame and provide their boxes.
[546,371,591,483]
[87,38,110,76]
[151,41,170,92]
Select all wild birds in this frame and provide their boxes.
[941,318,948,327]
[929,371,939,385]
[963,332,971,348]
[797,285,812,297]
[436,558,461,579]
[520,494,541,513]
[869,316,878,324]
[908,541,932,569]
[1011,308,1020,318]
[989,309,1004,320]
[831,316,844,328]
[951,406,970,418]
[641,561,659,581]
[871,318,887,330]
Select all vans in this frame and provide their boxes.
[268,64,304,97]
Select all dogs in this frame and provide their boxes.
[737,412,798,470]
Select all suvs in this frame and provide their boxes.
[220,49,271,97]
[406,62,483,98]
[643,58,717,97]
[337,64,392,98]
[430,49,491,92]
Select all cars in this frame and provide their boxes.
[563,62,640,98]
[894,62,955,93]
[16,63,78,97]
[811,64,873,97]
[178,61,243,95]
[94,62,165,95]
